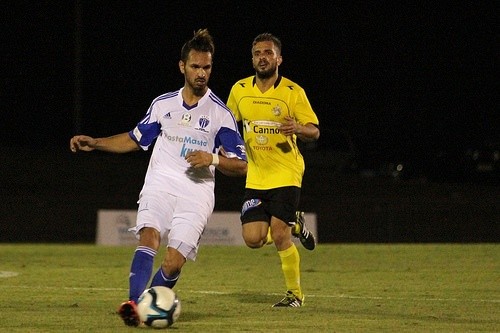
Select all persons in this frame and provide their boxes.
[70,29,249,327]
[227,34,320,309]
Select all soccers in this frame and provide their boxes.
[137,286,180,329]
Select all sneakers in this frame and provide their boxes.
[271,291,305,308]
[119,300,140,327]
[292,211,316,251]
